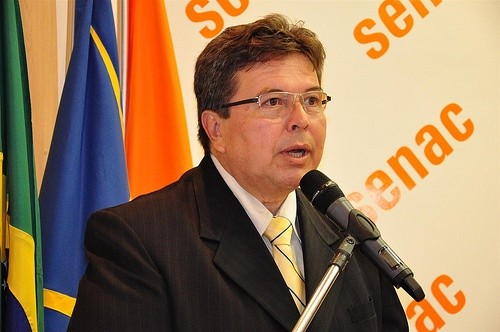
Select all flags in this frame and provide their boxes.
[0,0,45,332]
[43,0,130,332]
[119,0,193,202]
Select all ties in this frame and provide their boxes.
[263,215,309,316]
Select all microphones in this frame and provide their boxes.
[299,169,425,302]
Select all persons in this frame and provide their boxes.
[65,14,410,332]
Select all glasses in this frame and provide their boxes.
[214,89,331,114]
